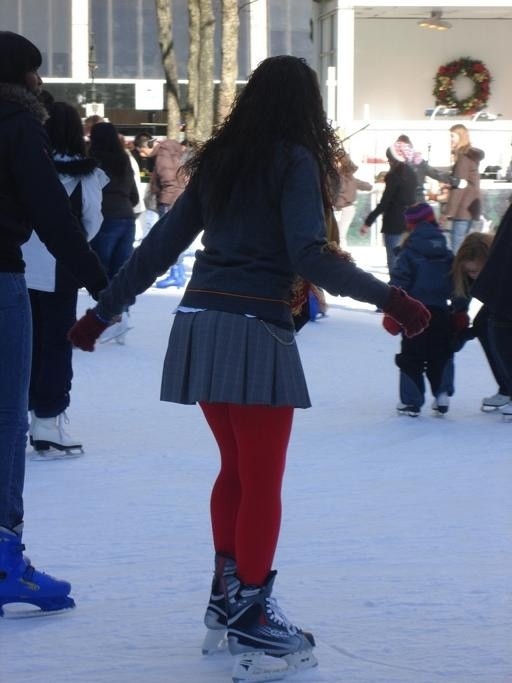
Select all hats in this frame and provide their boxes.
[405,201,439,230]
[388,134,427,166]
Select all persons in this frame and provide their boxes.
[134,131,190,292]
[0,30,112,621]
[330,123,512,421]
[19,90,143,459]
[65,55,431,683]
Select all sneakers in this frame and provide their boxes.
[98,311,136,343]
[28,411,84,451]
[397,400,421,413]
[430,391,450,413]
[481,392,512,414]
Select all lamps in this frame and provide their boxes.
[417,11,454,32]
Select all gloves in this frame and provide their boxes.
[441,216,454,231]
[359,224,370,236]
[447,308,469,329]
[66,308,115,353]
[449,330,472,353]
[382,284,432,339]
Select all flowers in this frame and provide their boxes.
[432,57,495,115]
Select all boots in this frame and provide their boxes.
[0,521,72,603]
[156,264,187,289]
[204,552,317,658]
